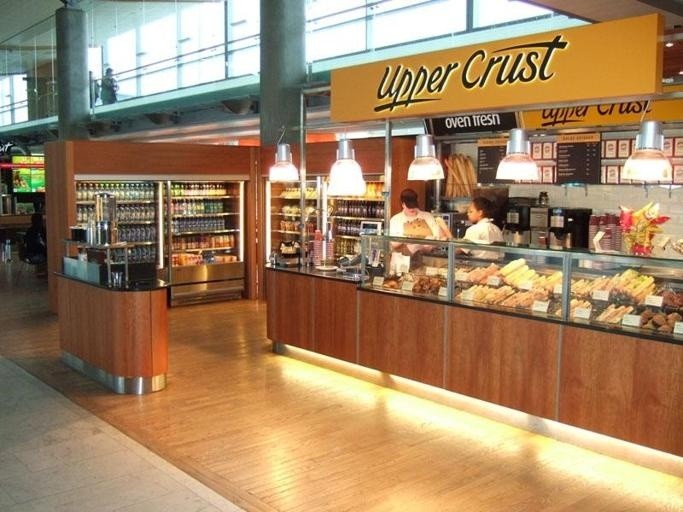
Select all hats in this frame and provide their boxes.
[401,189,420,208]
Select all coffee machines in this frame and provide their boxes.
[505,205,532,246]
[548,206,592,250]
[527,204,549,249]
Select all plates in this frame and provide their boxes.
[316,265,338,271]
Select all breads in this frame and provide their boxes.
[412,275,447,295]
[642,310,682,333]
[555,298,594,318]
[459,285,537,311]
[499,258,562,290]
[596,303,633,326]
[574,269,658,305]
[465,258,499,286]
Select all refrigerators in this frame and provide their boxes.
[263,174,387,265]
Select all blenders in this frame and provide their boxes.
[86,193,118,246]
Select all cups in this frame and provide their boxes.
[539,236,547,248]
[69,224,87,241]
[0,192,18,217]
[269,253,278,265]
[63,238,78,257]
[111,271,123,288]
[588,209,624,253]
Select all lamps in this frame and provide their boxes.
[269,144,298,183]
[621,119,673,181]
[326,140,367,196]
[404,133,445,182]
[494,129,543,182]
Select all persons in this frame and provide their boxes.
[101,68,119,105]
[390,188,448,270]
[90,71,100,108]
[435,197,506,262]
[24,213,46,264]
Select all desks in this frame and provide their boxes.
[52,268,170,395]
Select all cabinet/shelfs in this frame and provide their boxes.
[265,263,359,365]
[559,251,683,459]
[357,233,452,393]
[270,181,317,261]
[326,178,387,259]
[448,243,565,423]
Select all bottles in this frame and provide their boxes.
[93,183,99,200]
[165,233,235,251]
[204,217,209,231]
[196,218,200,231]
[131,224,136,241]
[217,199,224,213]
[200,217,204,231]
[120,183,125,200]
[280,186,318,234]
[76,183,83,200]
[174,184,178,196]
[184,217,187,232]
[140,224,146,241]
[149,183,155,199]
[150,224,156,241]
[126,225,131,242]
[140,183,145,200]
[140,204,145,222]
[175,200,179,214]
[187,201,192,214]
[207,200,210,213]
[188,184,191,195]
[182,200,188,214]
[163,184,166,196]
[199,185,203,196]
[192,201,196,214]
[87,183,94,201]
[170,199,174,214]
[191,184,195,195]
[145,184,149,199]
[222,184,227,195]
[184,184,189,196]
[179,218,184,232]
[192,218,196,232]
[135,225,141,242]
[177,184,180,195]
[206,184,210,195]
[144,203,150,222]
[204,201,208,213]
[125,205,129,221]
[164,220,168,233]
[209,200,214,212]
[145,224,151,241]
[134,183,140,200]
[179,201,184,214]
[195,184,200,196]
[196,201,201,214]
[212,185,216,195]
[135,204,140,221]
[539,191,550,208]
[4,239,12,262]
[115,183,120,201]
[87,206,92,223]
[221,216,225,229]
[77,206,82,222]
[187,218,192,232]
[119,204,124,222]
[216,216,221,229]
[216,184,220,195]
[105,183,110,192]
[113,247,152,263]
[171,184,174,196]
[162,249,216,267]
[82,183,88,201]
[209,185,213,195]
[110,183,115,193]
[164,200,168,215]
[180,184,185,196]
[213,199,218,213]
[129,183,135,201]
[208,217,212,231]
[82,205,88,223]
[116,205,120,221]
[331,181,385,265]
[121,225,126,242]
[174,218,180,232]
[150,204,156,220]
[171,220,174,232]
[220,185,223,195]
[212,217,217,230]
[200,201,205,214]
[202,185,206,196]
[129,204,134,222]
[99,183,105,192]
[124,183,130,201]
